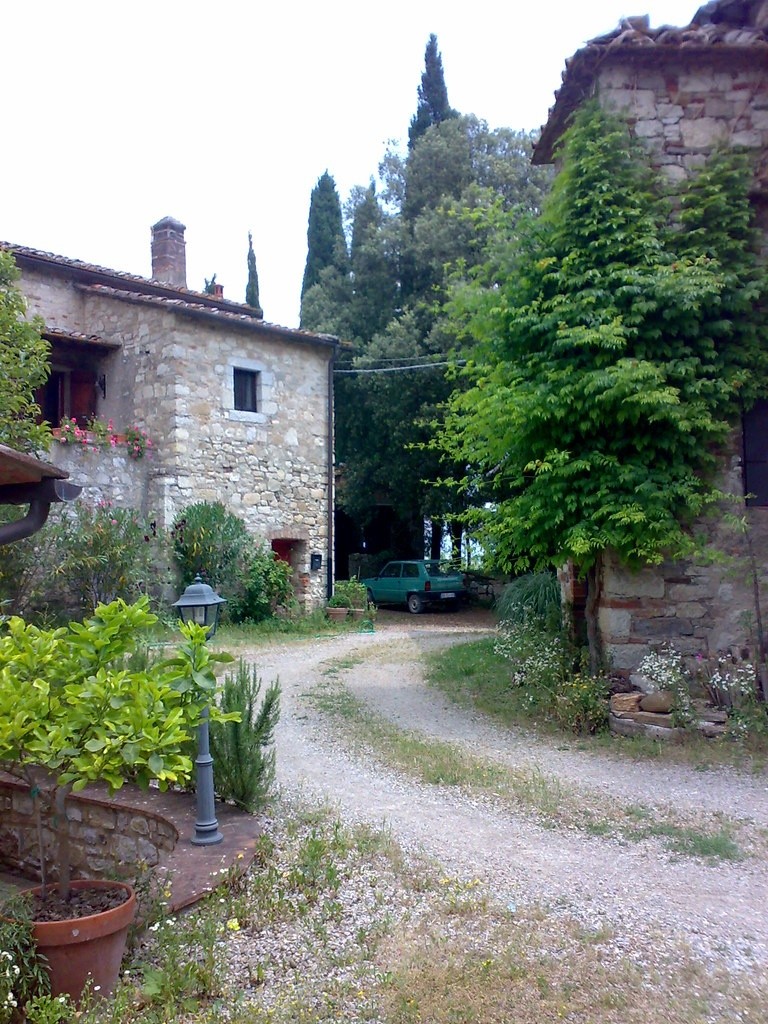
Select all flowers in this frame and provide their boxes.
[124,424,152,461]
[81,412,116,451]
[59,414,87,445]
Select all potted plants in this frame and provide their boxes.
[51,428,61,437]
[0,589,241,1009]
[352,600,365,620]
[325,595,351,623]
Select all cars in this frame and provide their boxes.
[359,559,471,614]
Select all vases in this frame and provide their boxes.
[85,431,94,440]
[116,434,126,441]
